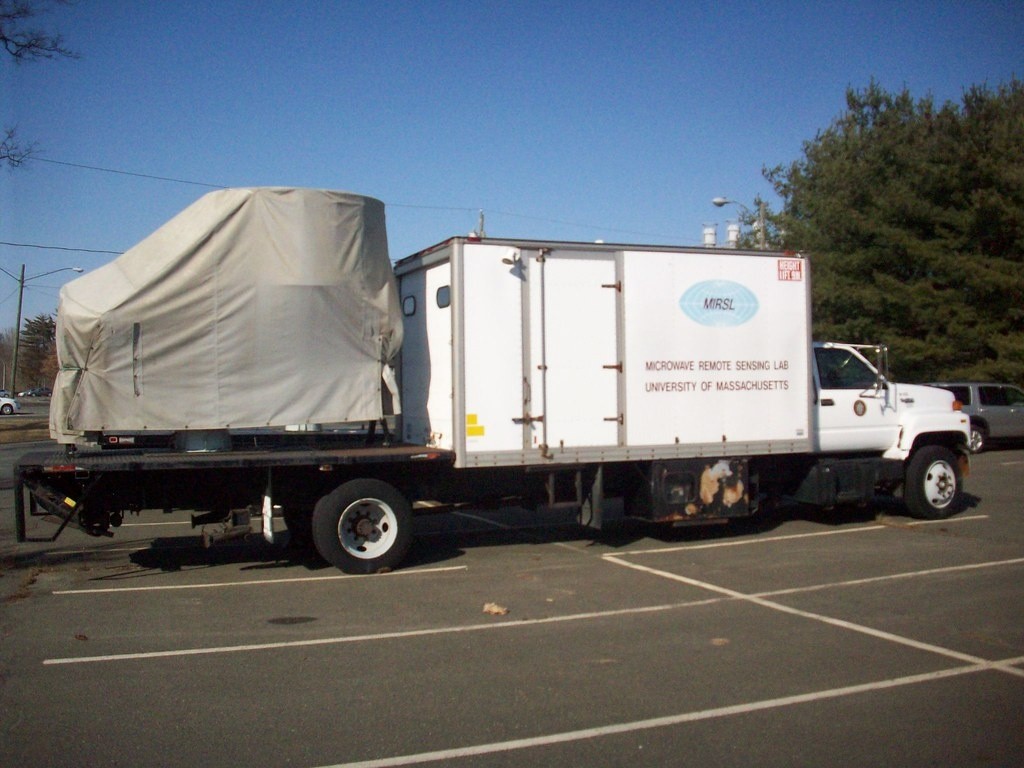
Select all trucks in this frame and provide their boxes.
[9,235,971,579]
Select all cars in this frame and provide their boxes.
[0,389,12,398]
[17,388,36,397]
[0,397,21,415]
[28,387,52,397]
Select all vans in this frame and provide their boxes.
[920,380,1024,455]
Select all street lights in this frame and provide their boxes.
[713,195,762,234]
